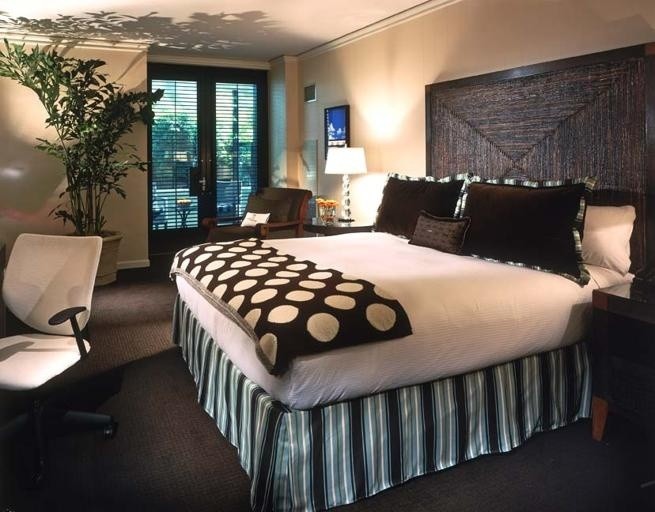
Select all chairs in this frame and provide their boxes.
[202,187,312,242]
[0,233,117,490]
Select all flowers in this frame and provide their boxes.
[316,198,339,208]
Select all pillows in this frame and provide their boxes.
[582,205,636,276]
[371,172,470,240]
[408,209,471,255]
[459,175,600,285]
[240,212,271,228]
[248,198,292,223]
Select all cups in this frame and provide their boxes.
[316,199,337,225]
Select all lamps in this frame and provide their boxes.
[324,147,368,222]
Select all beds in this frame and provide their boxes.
[169,41,654,512]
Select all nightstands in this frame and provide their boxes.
[303,217,375,236]
[591,280,654,442]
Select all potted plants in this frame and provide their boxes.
[0,37,167,287]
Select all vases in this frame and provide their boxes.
[319,206,337,222]
